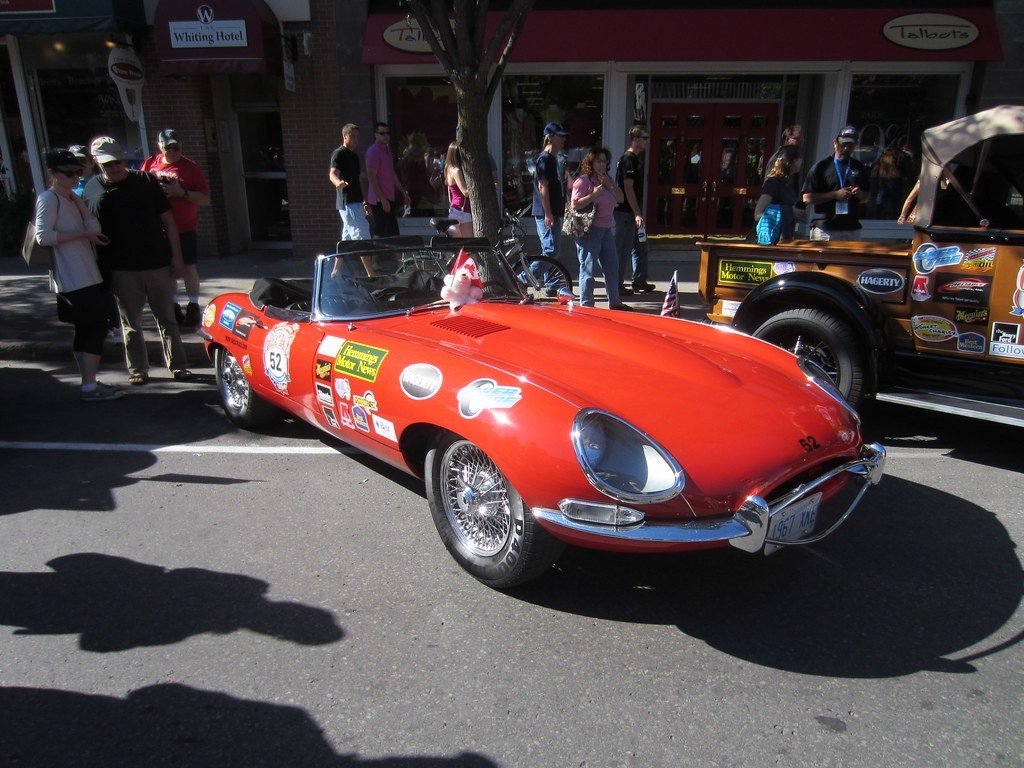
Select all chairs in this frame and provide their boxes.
[284,296,346,311]
[372,287,415,301]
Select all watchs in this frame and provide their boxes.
[183,190,188,200]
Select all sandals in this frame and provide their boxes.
[173,369,191,380]
[128,371,149,385]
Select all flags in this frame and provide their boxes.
[450,249,484,289]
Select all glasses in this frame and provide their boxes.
[791,136,804,140]
[103,160,122,167]
[164,145,180,152]
[56,168,83,178]
[377,131,391,136]
[639,136,649,140]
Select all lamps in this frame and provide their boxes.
[105,34,141,51]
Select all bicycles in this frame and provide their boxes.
[397,209,575,305]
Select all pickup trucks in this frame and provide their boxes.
[696,103,1024,429]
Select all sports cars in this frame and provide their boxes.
[197,241,886,588]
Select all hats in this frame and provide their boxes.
[91,137,125,164]
[837,125,858,146]
[69,145,93,160]
[544,122,571,137]
[46,151,86,171]
[158,129,180,147]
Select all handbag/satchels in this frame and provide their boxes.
[20,222,53,274]
[560,198,597,239]
[447,219,462,238]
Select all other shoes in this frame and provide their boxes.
[610,303,633,311]
[632,282,656,293]
[80,381,126,399]
[375,266,390,272]
[618,283,634,295]
[174,303,201,331]
[113,327,124,347]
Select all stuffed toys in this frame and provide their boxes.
[441,267,483,311]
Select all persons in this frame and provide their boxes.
[444,142,478,271]
[35,149,125,401]
[571,148,633,312]
[517,122,569,297]
[488,153,499,189]
[754,125,921,242]
[330,124,375,278]
[366,122,411,267]
[141,130,211,328]
[82,136,191,384]
[396,135,449,209]
[69,146,99,199]
[612,126,655,295]
[657,140,763,233]
